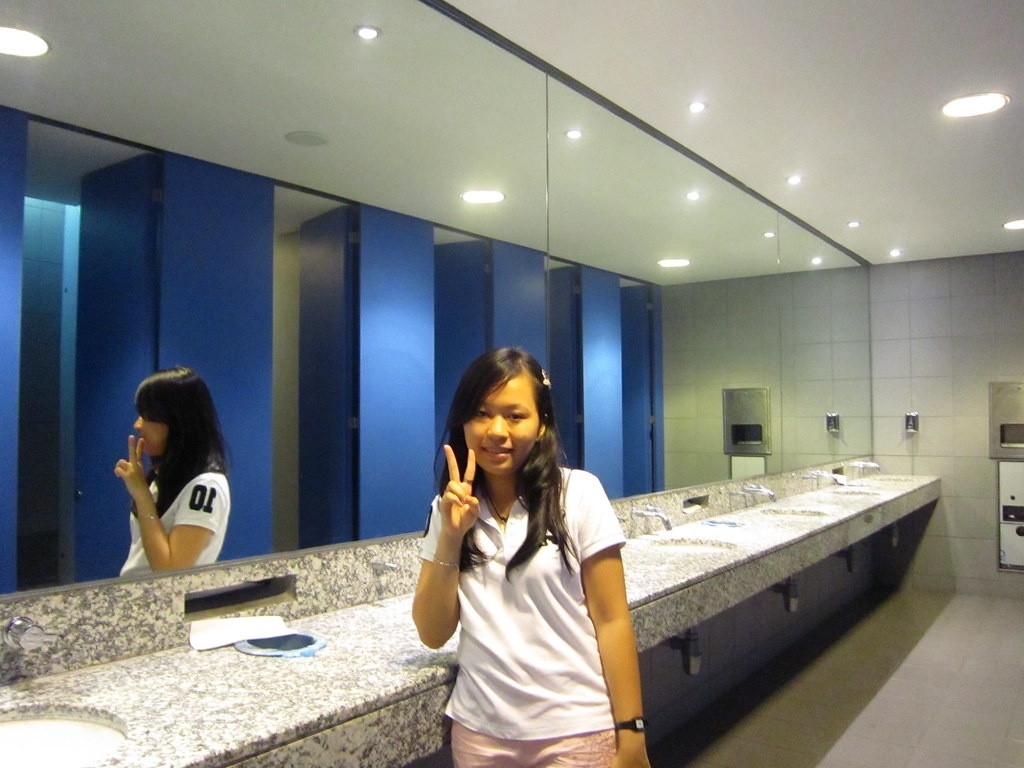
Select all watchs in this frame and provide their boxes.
[616,716,648,732]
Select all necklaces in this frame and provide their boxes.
[484,488,511,524]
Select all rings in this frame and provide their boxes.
[464,479,473,484]
[135,458,143,461]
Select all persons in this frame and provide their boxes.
[114,363,233,577]
[409,346,651,767]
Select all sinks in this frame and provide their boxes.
[879,474,924,485]
[0,700,130,767]
[829,488,883,500]
[762,505,831,521]
[646,537,740,559]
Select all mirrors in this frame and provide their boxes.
[0,0,871,594]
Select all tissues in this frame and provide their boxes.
[831,467,852,484]
[681,495,709,515]
[986,380,1024,461]
[721,385,773,456]
[184,580,274,614]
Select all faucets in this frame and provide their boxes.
[628,503,674,534]
[743,482,780,505]
[855,461,881,474]
[810,469,841,486]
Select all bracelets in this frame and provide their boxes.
[138,513,160,520]
[432,555,461,570]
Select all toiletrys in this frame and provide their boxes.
[825,412,840,433]
[905,412,919,435]
